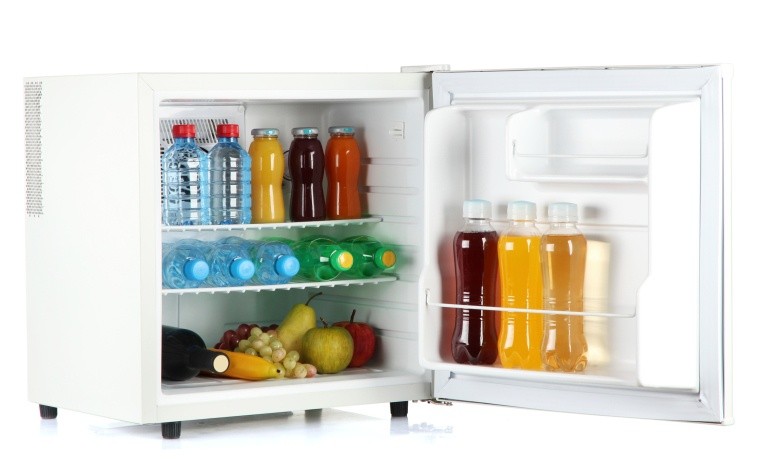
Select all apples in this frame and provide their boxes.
[302,317,354,373]
[332,309,376,368]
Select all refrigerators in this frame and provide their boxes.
[25,65,735,440]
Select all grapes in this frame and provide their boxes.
[213,323,317,378]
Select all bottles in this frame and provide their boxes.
[220,236,300,285]
[249,128,285,224]
[325,126,362,220]
[208,124,251,225]
[497,202,544,369]
[262,235,354,283]
[162,325,230,381]
[452,199,499,366]
[539,202,589,374]
[162,243,209,289]
[307,236,397,280]
[179,239,255,287]
[288,127,325,222]
[162,124,210,225]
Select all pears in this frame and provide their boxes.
[275,292,323,361]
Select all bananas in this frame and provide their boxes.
[206,348,280,380]
